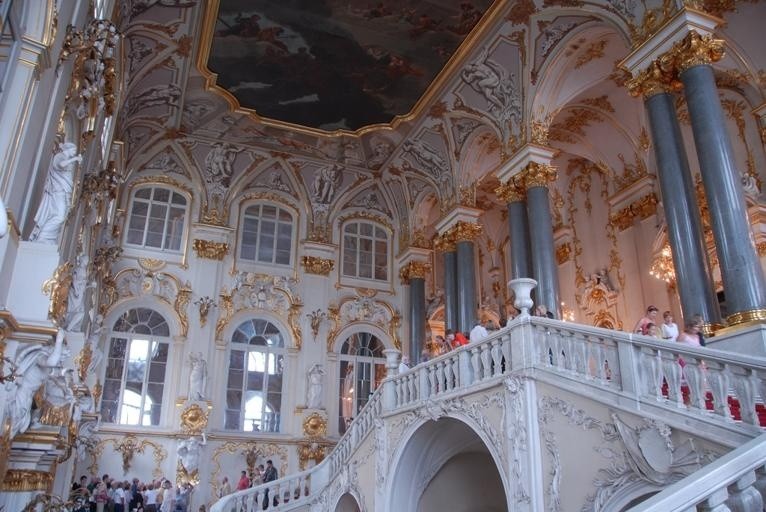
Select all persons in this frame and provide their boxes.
[29,141,81,243]
[128,87,181,113]
[461,46,507,112]
[403,137,445,172]
[318,163,344,203]
[206,143,244,177]
[633,306,711,402]
[399,305,554,392]
[71,460,278,512]
[188,352,207,401]
[308,366,326,409]
[0,255,106,441]
[177,432,207,472]
